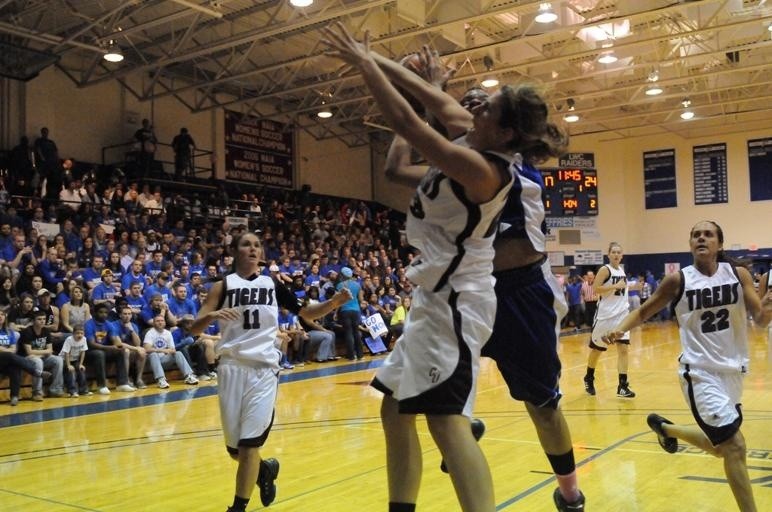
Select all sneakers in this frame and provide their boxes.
[184,374,198,384]
[279,365,284,371]
[290,359,304,367]
[616,383,635,397]
[135,378,148,389]
[97,386,111,395]
[280,361,294,369]
[584,375,595,395]
[10,395,18,405]
[36,371,52,380]
[256,459,278,508]
[207,372,217,381]
[71,392,79,398]
[32,393,43,401]
[553,487,586,512]
[80,391,94,396]
[647,414,677,453]
[157,378,170,388]
[128,382,137,390]
[48,393,71,398]
[441,419,484,473]
[116,385,135,392]
[199,375,211,381]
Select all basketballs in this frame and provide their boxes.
[391,53,448,110]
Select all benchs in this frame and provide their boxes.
[0,358,198,404]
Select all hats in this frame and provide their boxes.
[101,269,114,281]
[37,288,50,298]
[341,266,353,278]
[370,257,379,269]
[269,265,280,272]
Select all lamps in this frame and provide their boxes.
[317,84,336,119]
[536,0,696,122]
[103,24,125,62]
[481,55,499,88]
[562,98,580,124]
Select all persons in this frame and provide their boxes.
[133,119,158,154]
[0,127,59,195]
[383,40,585,511]
[626,269,670,320]
[171,127,195,176]
[0,160,420,405]
[317,18,573,511]
[185,229,355,512]
[564,271,598,331]
[599,218,770,511]
